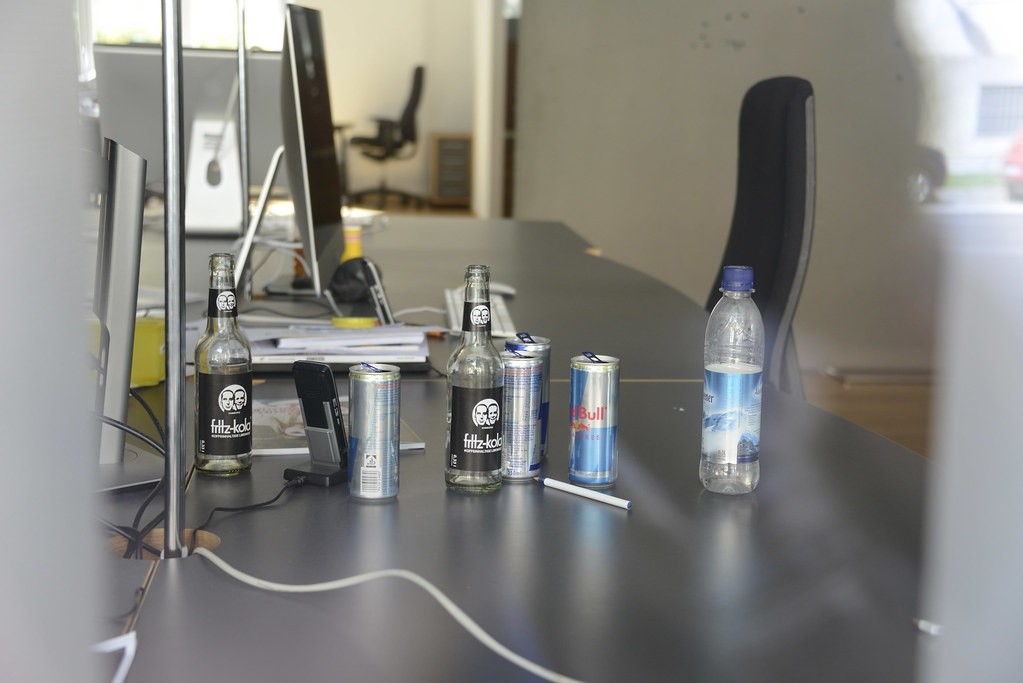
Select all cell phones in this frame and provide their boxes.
[292,360,349,463]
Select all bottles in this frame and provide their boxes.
[698,265,765,494]
[193,252,254,478]
[443,264,505,497]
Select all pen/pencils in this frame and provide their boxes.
[532,475,632,512]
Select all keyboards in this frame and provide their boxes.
[443,288,517,338]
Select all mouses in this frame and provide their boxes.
[454,282,516,295]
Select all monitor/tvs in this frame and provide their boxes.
[233,3,346,299]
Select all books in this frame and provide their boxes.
[250,331,430,363]
[252,397,425,456]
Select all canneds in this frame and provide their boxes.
[499,331,552,481]
[347,361,401,502]
[570,351,620,488]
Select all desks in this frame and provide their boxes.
[97,219,940,683]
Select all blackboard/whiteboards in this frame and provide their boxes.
[430,133,472,204]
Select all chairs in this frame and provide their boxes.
[704,74,819,405]
[350,65,428,211]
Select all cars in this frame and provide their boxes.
[1001,121,1023,202]
[909,142,949,207]
